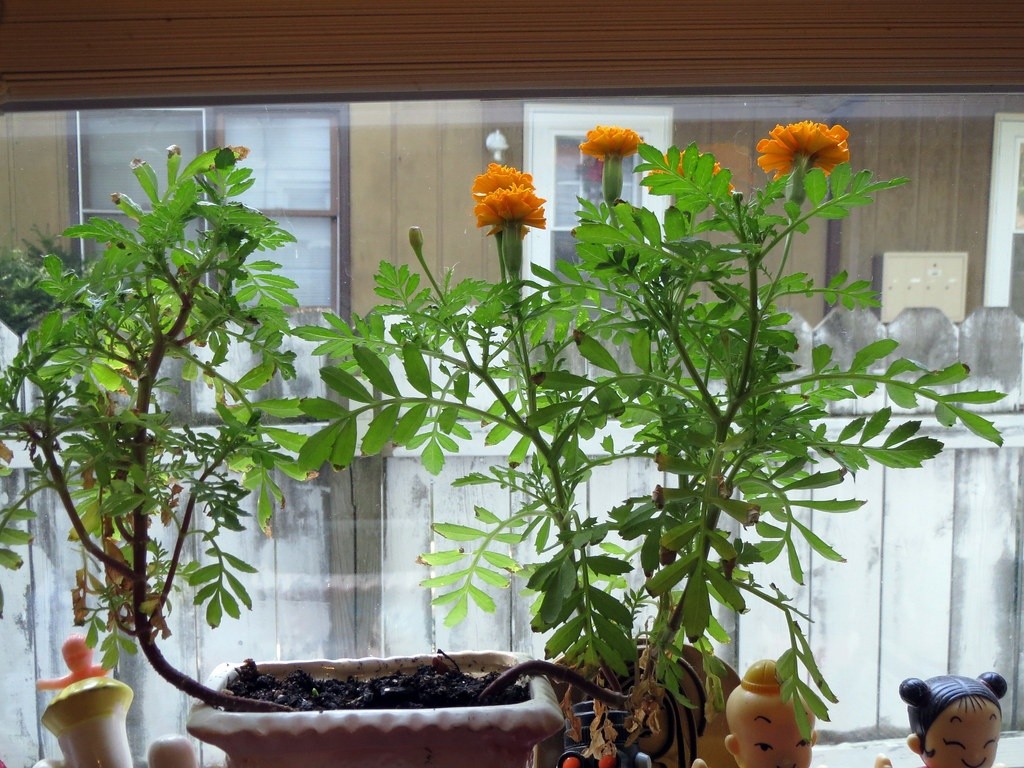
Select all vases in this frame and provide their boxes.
[185,649,565,768]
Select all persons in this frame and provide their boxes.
[691,659,829,768]
[873,672,1009,768]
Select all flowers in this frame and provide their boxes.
[0,121,1019,713]
